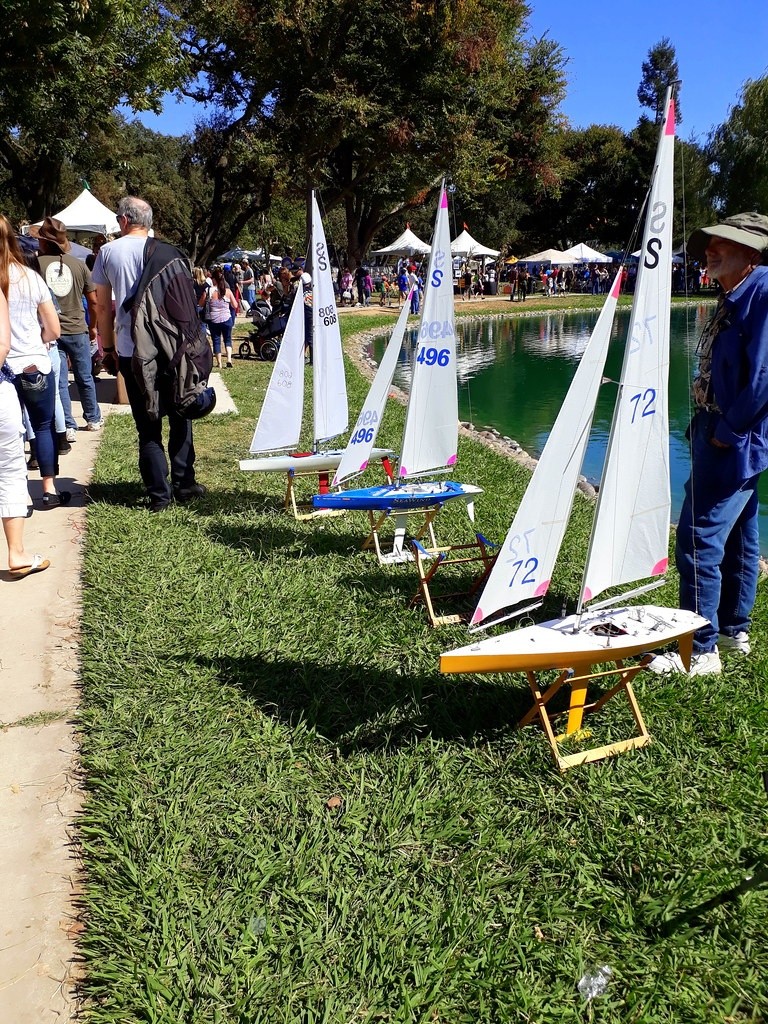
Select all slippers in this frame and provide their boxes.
[8,554,50,579]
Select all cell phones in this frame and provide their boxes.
[101,353,119,377]
[23,364,38,373]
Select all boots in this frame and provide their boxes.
[27,438,39,470]
[57,433,71,455]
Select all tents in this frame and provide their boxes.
[215,245,282,263]
[371,229,432,266]
[517,248,583,268]
[20,189,155,239]
[450,230,500,259]
[564,242,613,263]
[631,249,683,263]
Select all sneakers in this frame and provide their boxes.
[88,419,104,431]
[66,429,76,441]
[27,506,33,517]
[718,632,751,657]
[648,644,722,677]
[43,491,71,506]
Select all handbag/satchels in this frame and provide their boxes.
[201,286,211,323]
[343,288,351,298]
[241,296,250,311]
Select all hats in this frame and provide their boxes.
[700,212,768,252]
[410,266,417,271]
[29,216,71,253]
[381,276,387,281]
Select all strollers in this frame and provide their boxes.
[240,298,294,362]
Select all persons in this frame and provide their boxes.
[506,264,575,302]
[0,286,50,578]
[263,264,313,365]
[672,262,720,295]
[573,262,638,296]
[474,275,486,299]
[379,276,390,306]
[394,256,425,315]
[646,209,768,677]
[460,269,472,301]
[0,197,208,511]
[330,261,373,306]
[190,257,274,367]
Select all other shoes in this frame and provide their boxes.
[93,377,101,383]
[174,483,206,504]
[226,360,233,368]
[216,365,223,369]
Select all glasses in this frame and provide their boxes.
[116,215,121,223]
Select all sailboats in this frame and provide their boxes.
[440,82,711,675]
[313,177,484,509]
[238,188,396,472]
